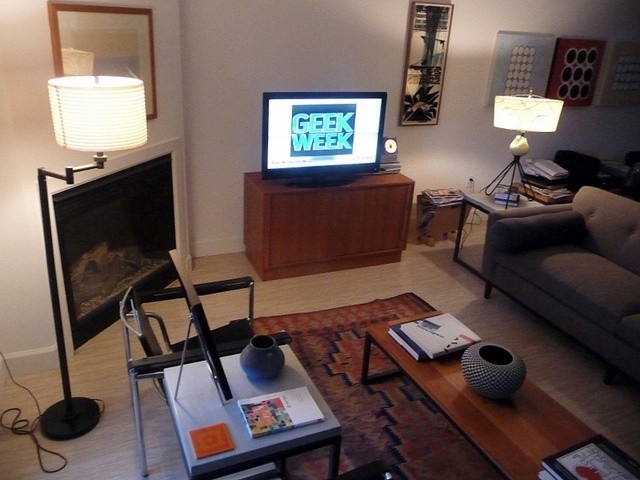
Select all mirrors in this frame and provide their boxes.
[47,3,157,120]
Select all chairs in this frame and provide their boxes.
[609,151,640,195]
[119,275,292,478]
[554,150,600,193]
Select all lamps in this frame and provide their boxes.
[485,89,564,210]
[37,76,148,441]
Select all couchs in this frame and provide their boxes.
[481,185,640,380]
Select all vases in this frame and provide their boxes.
[240,333,284,382]
[461,341,526,400]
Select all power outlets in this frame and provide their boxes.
[465,176,475,189]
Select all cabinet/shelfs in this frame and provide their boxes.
[243,172,415,281]
[416,194,471,247]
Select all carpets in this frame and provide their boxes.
[248,292,506,479]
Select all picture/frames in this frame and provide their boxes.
[399,0,455,126]
[168,248,234,406]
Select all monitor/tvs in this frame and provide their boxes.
[261,92,387,188]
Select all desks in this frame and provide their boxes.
[600,160,639,177]
[453,193,547,280]
[163,344,342,479]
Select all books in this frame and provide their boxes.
[520,157,574,200]
[388,314,446,360]
[537,469,557,480]
[236,386,326,438]
[400,313,482,359]
[540,433,640,480]
[492,192,520,207]
[422,187,465,207]
